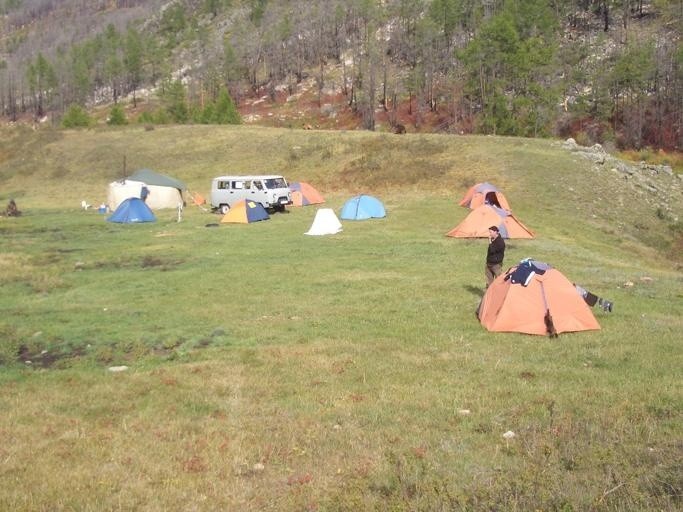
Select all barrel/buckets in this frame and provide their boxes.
[99,205,106,214]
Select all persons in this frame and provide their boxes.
[4,198,17,216]
[486,226,505,289]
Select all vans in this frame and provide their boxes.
[209,175,294,216]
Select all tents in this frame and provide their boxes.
[476,258,600,336]
[108,197,156,223]
[458,180,510,210]
[288,181,325,207]
[304,208,342,235]
[445,203,536,239]
[110,168,186,209]
[220,199,270,224]
[340,193,386,220]
